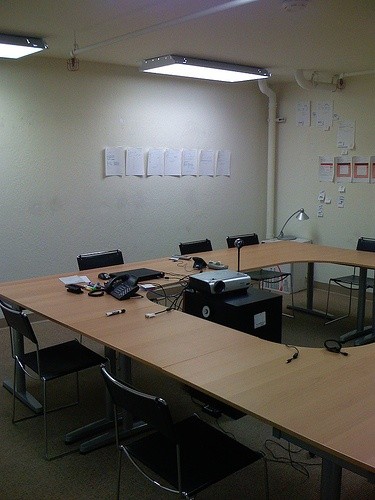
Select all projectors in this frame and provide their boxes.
[188,270,251,294]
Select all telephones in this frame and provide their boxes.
[104,273,140,300]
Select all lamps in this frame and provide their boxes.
[0,35,49,61]
[277,208,309,240]
[139,55,271,85]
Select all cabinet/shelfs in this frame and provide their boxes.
[260,237,311,294]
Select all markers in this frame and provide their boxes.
[106,309,126,317]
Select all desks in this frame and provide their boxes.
[0,241,375,500]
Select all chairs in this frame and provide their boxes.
[76,249,124,271]
[0,300,109,459]
[226,233,295,319]
[99,363,268,500]
[178,238,213,256]
[323,237,375,327]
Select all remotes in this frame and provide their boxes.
[172,255,191,260]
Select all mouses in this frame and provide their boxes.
[98,273,110,280]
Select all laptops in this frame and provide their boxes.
[110,268,164,282]
